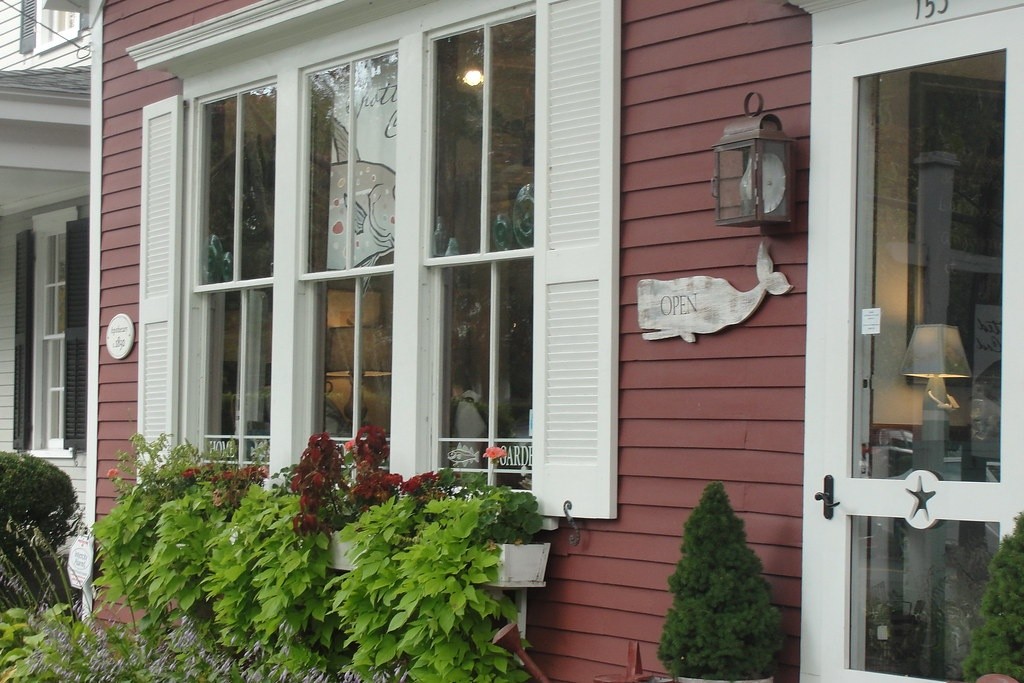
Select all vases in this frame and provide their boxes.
[327,530,552,591]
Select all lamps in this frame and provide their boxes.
[326,327,393,437]
[901,323,972,439]
[712,93,792,227]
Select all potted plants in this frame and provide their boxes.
[654,482,786,683]
[0,449,88,614]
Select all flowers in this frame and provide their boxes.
[106,430,544,543]
